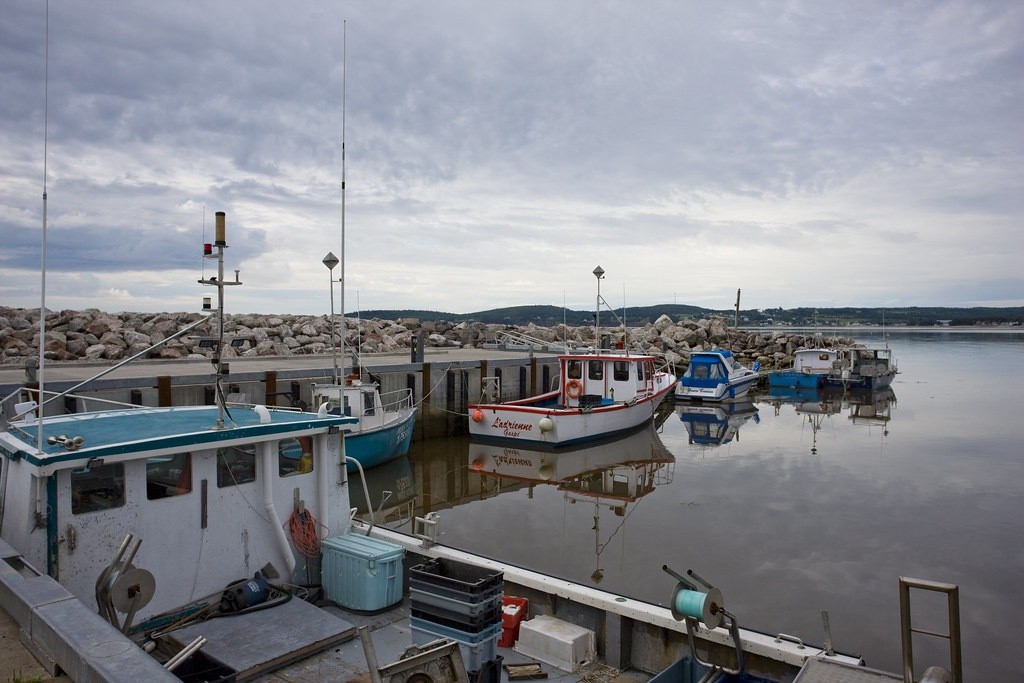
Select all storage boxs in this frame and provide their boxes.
[646,653,783,683]
[498,594,528,646]
[407,556,504,683]
[319,531,405,614]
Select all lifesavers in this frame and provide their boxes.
[565,379,583,398]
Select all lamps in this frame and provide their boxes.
[328,424,339,435]
[86,457,105,469]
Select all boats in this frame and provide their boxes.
[0,0,967,683]
[768,312,845,389]
[768,387,849,455]
[675,397,761,459]
[827,309,902,389]
[468,417,677,584]
[467,264,678,448]
[228,20,418,475]
[675,326,760,402]
[847,386,898,460]
